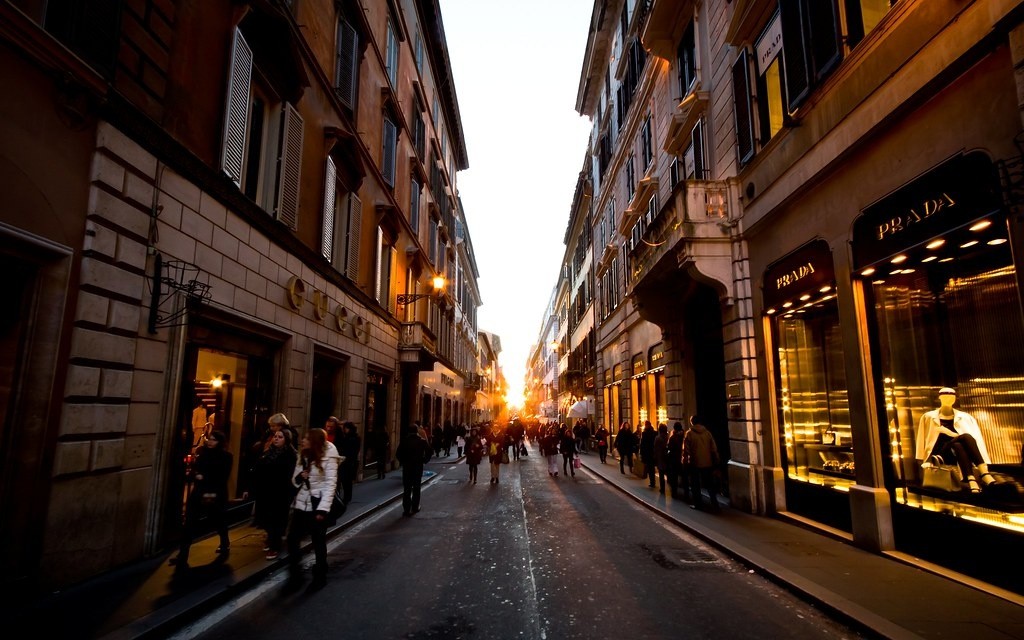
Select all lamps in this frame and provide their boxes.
[396,270,446,305]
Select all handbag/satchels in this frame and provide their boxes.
[311,493,346,524]
[521,441,528,456]
[501,453,509,463]
[574,459,580,468]
[924,456,962,492]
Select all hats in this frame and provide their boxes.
[268,414,289,424]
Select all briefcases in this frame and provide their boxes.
[634,460,648,478]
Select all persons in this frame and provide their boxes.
[413,414,643,485]
[281,427,346,594]
[342,421,361,504]
[322,415,346,473]
[170,429,234,562]
[654,423,677,498]
[242,414,300,559]
[915,387,1002,495]
[639,420,660,487]
[395,423,433,516]
[371,424,390,480]
[666,421,691,499]
[681,414,721,512]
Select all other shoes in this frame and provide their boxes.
[982,473,999,486]
[216,545,230,551]
[969,479,981,495]
[496,478,498,483]
[169,552,189,564]
[266,552,277,559]
[490,478,495,483]
